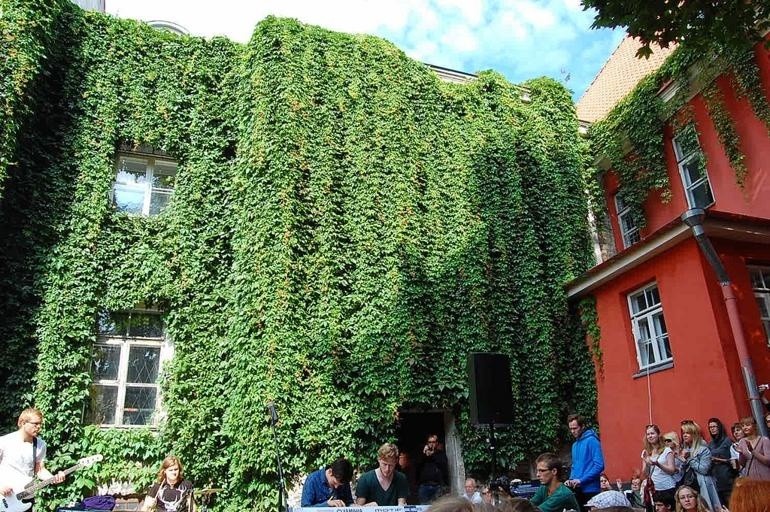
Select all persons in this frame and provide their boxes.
[418,433,451,505]
[564,416,604,510]
[139,454,196,512]
[428,475,534,510]
[300,458,354,507]
[586,470,643,510]
[531,453,580,510]
[395,448,416,505]
[355,442,408,507]
[0,408,65,511]
[640,416,768,511]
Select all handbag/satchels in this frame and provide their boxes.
[638,476,656,508]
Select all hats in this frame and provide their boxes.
[584,490,633,509]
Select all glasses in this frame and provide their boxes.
[679,420,694,425]
[427,440,437,444]
[664,439,672,442]
[534,468,553,473]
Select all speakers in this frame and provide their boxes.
[469,352,513,427]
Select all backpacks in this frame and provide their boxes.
[678,462,701,494]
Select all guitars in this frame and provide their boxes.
[1,454,104,512]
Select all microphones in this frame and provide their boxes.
[267,402,279,422]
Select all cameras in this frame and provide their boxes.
[492,475,510,494]
